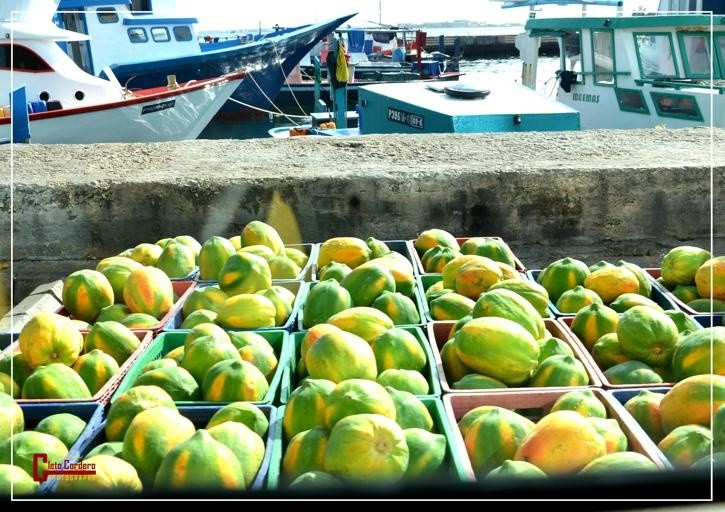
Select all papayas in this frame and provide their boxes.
[0,217,725,499]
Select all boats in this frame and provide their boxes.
[0,25,243,139]
[49,0,359,109]
[500,0,725,130]
[275,27,458,104]
